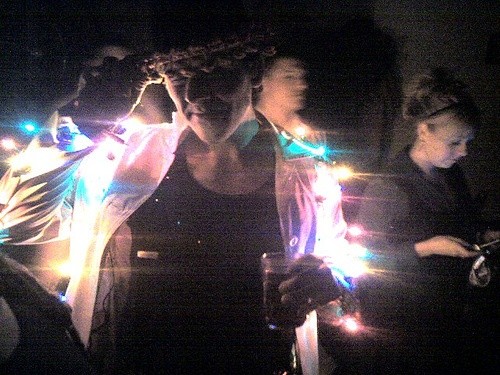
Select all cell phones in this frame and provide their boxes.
[469,239,499,251]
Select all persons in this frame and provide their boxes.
[0,8,341,375]
[253,50,343,375]
[359,66,500,375]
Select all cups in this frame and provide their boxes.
[260,251,307,331]
